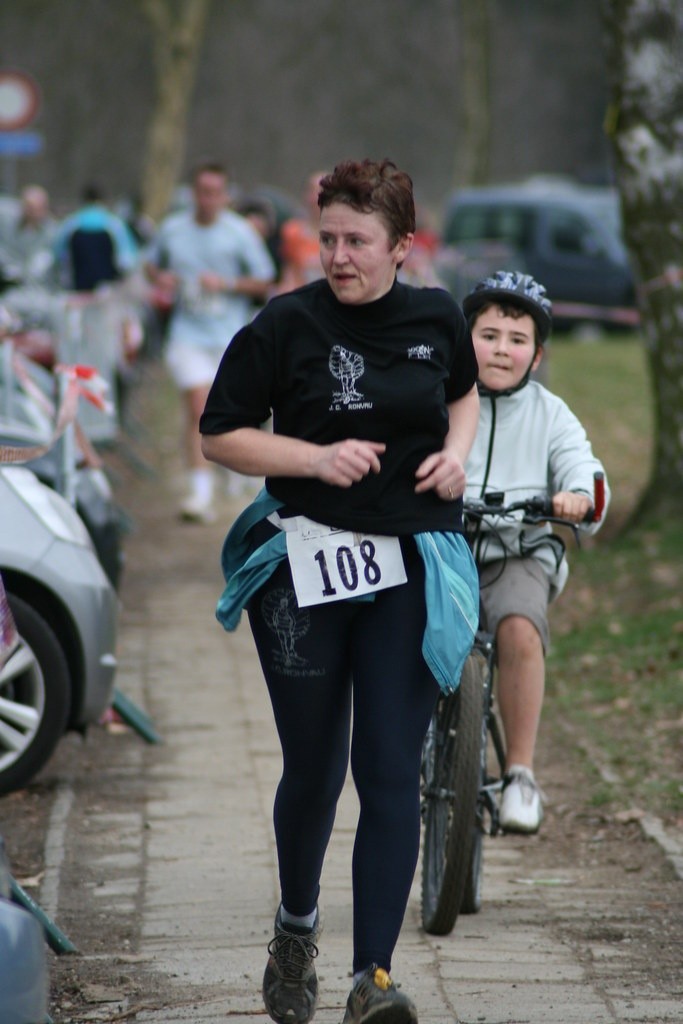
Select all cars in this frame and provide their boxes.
[442,173,637,337]
[1,316,168,1024]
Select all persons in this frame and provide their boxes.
[462,271,612,832]
[199,156,480,1024]
[15,164,442,526]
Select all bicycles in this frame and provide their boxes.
[410,480,597,938]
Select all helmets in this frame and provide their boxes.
[464,270,552,342]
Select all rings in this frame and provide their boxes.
[448,486,451,494]
[451,492,453,499]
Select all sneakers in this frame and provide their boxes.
[262,900,324,1024]
[342,963,418,1024]
[498,772,546,833]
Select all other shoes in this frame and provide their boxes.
[178,491,215,523]
